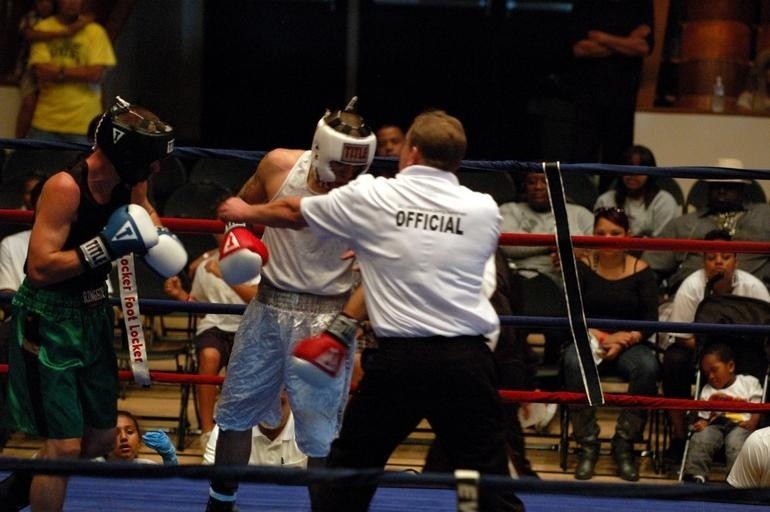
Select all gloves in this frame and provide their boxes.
[290,313,360,389]
[219,221,269,287]
[143,227,189,281]
[79,203,159,271]
[141,429,179,467]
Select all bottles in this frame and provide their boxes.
[711,75,726,112]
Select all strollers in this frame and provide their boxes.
[680,274,769,489]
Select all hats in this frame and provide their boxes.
[703,157,752,184]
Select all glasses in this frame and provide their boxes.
[594,206,626,218]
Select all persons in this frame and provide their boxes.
[5,96,191,510]
[216,108,526,511]
[1,0,770,488]
[202,106,379,511]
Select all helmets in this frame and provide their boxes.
[93,96,177,183]
[310,108,377,190]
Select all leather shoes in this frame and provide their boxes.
[575,439,600,480]
[610,436,640,482]
